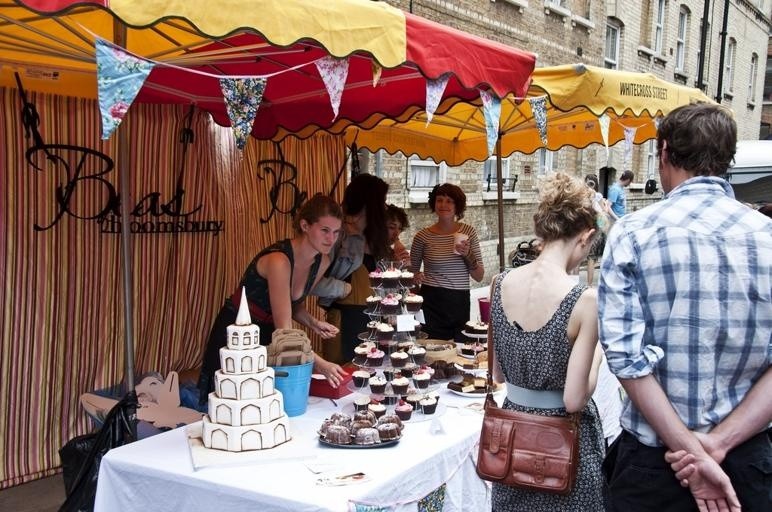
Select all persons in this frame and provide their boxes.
[598,103,771,511]
[196,191,345,405]
[309,173,391,365]
[571,170,634,286]
[341,203,410,363]
[474,170,606,512]
[410,183,484,343]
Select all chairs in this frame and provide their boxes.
[79,373,170,446]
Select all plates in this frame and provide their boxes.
[319,437,402,448]
[448,387,501,397]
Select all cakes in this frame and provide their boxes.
[316,264,502,445]
[202,284,292,453]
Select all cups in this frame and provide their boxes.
[452,232,469,256]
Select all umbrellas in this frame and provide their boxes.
[0,1,537,401]
[322,59,718,263]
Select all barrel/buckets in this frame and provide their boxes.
[271,361,313,416]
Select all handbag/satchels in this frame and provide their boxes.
[475,396,584,498]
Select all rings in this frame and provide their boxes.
[330,374,334,378]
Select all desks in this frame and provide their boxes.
[102,356,628,511]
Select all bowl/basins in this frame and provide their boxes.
[417,340,457,366]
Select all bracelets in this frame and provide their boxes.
[463,248,478,271]
